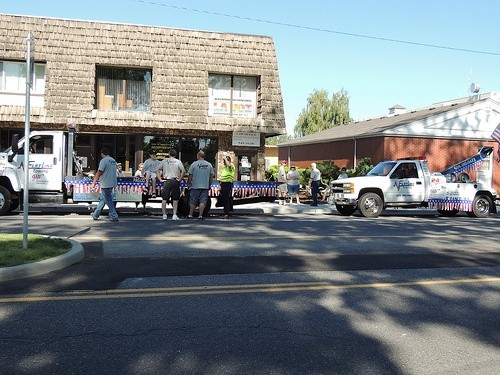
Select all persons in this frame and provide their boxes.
[308,163,321,206]
[219,156,236,219]
[156,149,185,220]
[90,147,119,222]
[135,163,146,211]
[337,167,348,180]
[141,148,162,198]
[117,163,125,178]
[188,151,214,219]
[278,160,289,205]
[286,164,301,205]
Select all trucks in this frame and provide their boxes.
[0,130,277,218]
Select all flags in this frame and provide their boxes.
[491,123,500,163]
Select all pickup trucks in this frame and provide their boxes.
[326,146,497,217]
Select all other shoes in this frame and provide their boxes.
[91,212,98,220]
[143,189,148,192]
[172,215,179,220]
[279,201,287,205]
[163,214,167,220]
[219,215,228,219]
[152,193,156,197]
[110,219,119,222]
[310,204,317,206]
[187,215,193,219]
[198,216,202,220]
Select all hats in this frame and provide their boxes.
[149,149,156,154]
